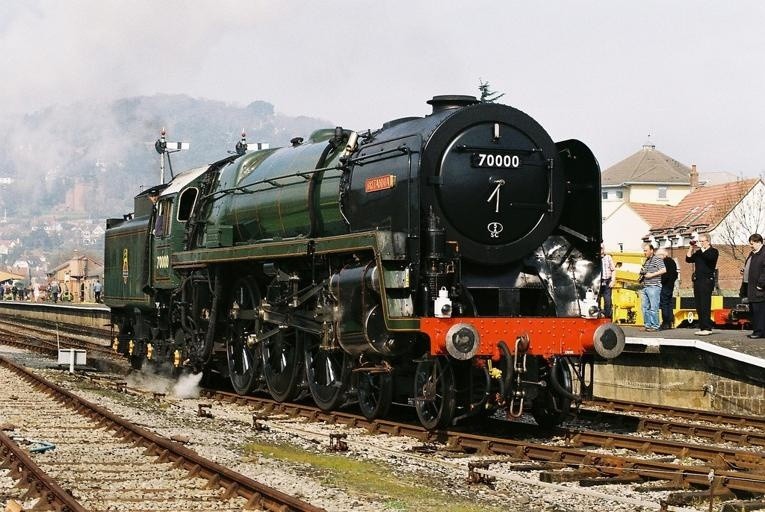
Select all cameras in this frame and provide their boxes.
[637,270,648,283]
[689,240,697,247]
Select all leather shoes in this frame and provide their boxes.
[748,330,764,338]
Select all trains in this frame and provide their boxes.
[103,93,626,435]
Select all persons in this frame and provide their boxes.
[739,233,765,339]
[588,243,616,331]
[686,233,719,336]
[32,281,41,303]
[49,280,60,303]
[655,248,678,331]
[0,284,34,301]
[93,281,102,303]
[45,284,52,301]
[638,244,667,332]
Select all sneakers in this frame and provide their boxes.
[695,328,712,336]
[640,323,672,332]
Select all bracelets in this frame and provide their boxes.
[610,280,613,282]
[699,245,703,249]
